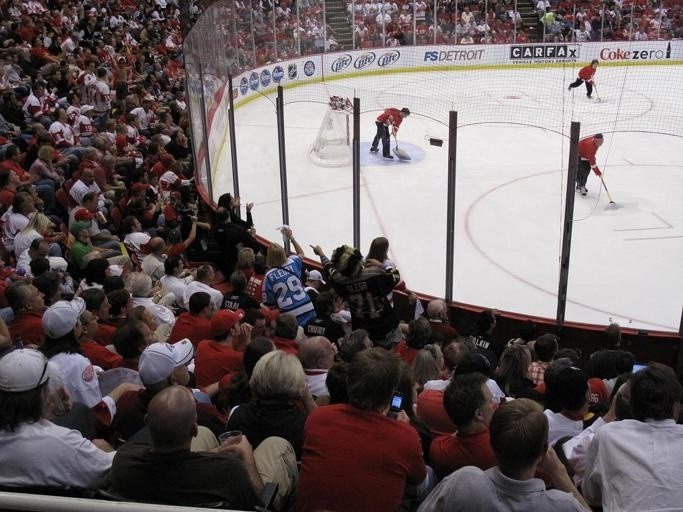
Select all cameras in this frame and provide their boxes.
[632,364,648,373]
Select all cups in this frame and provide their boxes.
[218,430,243,459]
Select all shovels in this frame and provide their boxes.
[388,118,411,161]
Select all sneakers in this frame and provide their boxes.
[383,155,393,158]
[370,148,379,151]
[576,185,588,196]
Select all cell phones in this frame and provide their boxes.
[387,391,405,420]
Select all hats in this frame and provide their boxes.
[210,309,245,335]
[306,269,326,285]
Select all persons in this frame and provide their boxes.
[0,1,682,510]
